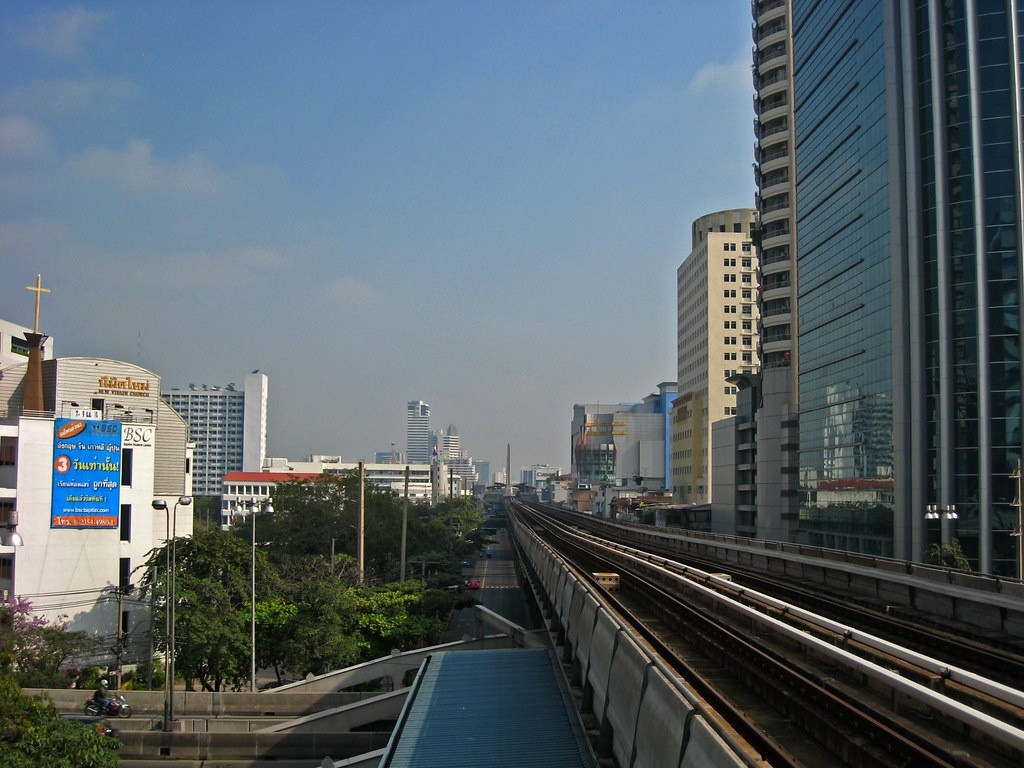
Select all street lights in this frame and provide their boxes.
[152,494,192,733]
[234,495,275,694]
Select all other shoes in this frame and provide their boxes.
[100,707,106,709]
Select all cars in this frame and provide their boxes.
[467,578,480,591]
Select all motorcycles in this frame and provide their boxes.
[84,692,132,719]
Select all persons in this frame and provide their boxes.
[94,679,116,710]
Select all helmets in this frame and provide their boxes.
[101,680,108,688]
